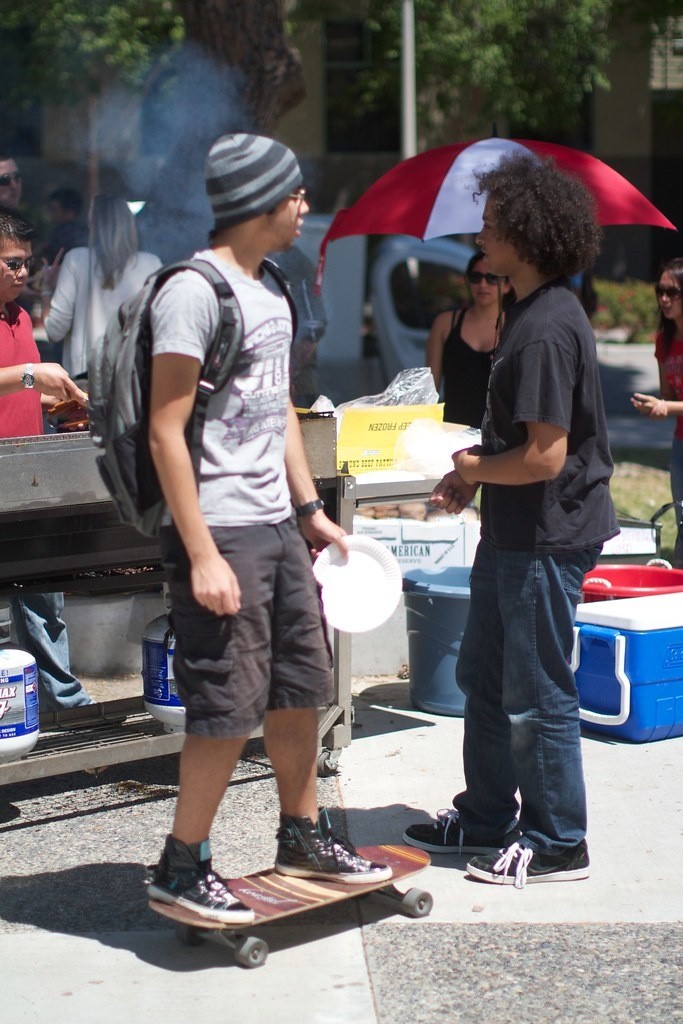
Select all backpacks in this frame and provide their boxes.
[87,258,297,537]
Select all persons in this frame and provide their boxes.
[262,244,327,383]
[406,260,464,330]
[402,147,621,888]
[0,152,97,708]
[147,133,393,924]
[40,192,164,394]
[426,252,511,430]
[568,262,592,320]
[630,254,683,571]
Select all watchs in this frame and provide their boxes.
[20,363,35,388]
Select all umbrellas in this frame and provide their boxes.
[313,119,677,299]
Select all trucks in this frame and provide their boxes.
[294,217,479,410]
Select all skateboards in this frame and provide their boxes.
[145,842,437,969]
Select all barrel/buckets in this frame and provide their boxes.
[579,559,683,602]
[402,565,473,717]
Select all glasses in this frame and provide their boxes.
[288,188,305,207]
[469,271,504,285]
[1,257,34,270]
[0,171,23,186]
[655,286,683,300]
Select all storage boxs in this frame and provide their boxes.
[294,401,445,476]
[567,592,683,742]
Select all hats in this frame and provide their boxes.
[203,132,303,228]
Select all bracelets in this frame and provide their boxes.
[39,290,53,298]
[296,498,324,517]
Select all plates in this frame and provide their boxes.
[312,535,403,633]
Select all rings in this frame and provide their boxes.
[69,385,78,392]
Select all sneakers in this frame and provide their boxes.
[402,808,507,856]
[274,806,393,885]
[147,834,255,923]
[466,841,591,888]
